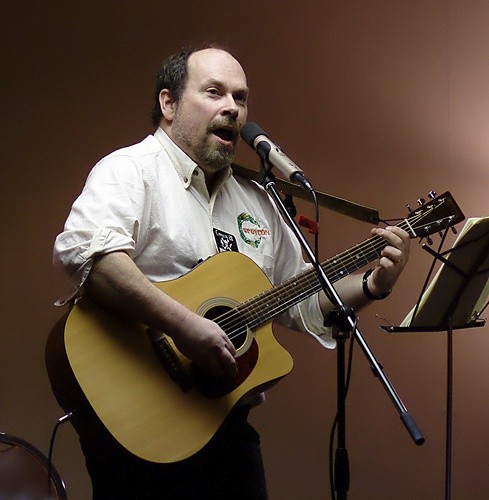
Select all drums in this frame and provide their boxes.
[0,431,67,500]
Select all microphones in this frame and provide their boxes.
[239,122,315,193]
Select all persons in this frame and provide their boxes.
[51,45,411,500]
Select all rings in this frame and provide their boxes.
[393,259,403,263]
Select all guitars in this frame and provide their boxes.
[43,187,465,483]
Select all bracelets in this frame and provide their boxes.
[362,268,393,301]
